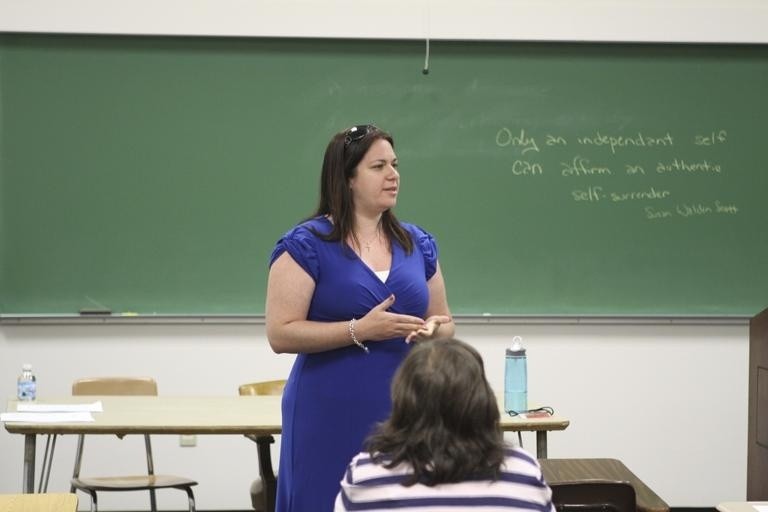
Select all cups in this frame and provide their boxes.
[504,336,528,413]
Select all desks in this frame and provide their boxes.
[539,457,662,509]
[4,391,567,501]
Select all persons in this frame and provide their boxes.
[264,124,457,512]
[331,336,554,510]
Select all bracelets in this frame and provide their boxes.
[347,318,371,356]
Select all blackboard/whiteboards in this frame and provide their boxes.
[1,29,766,324]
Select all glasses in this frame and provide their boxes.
[342,124,378,147]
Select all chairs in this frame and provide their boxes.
[67,376,198,511]
[0,492,79,511]
[549,480,637,508]
[236,381,296,510]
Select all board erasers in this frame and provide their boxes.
[80,308,111,315]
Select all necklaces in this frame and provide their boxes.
[347,225,379,252]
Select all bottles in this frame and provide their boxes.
[17,363,37,405]
